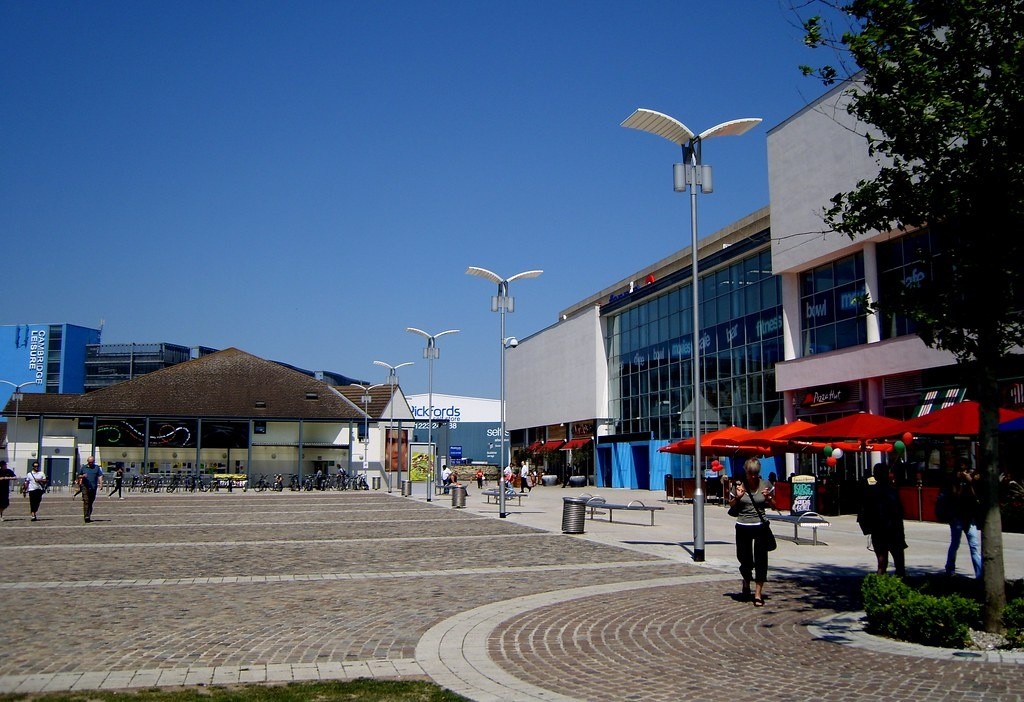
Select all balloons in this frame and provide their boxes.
[895,440,904,453]
[823,444,843,466]
[711,460,724,472]
[902,431,913,445]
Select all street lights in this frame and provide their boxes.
[350,383,385,484]
[465,266,544,519]
[405,327,460,501]
[372,360,414,493]
[619,108,764,561]
[0,379,38,491]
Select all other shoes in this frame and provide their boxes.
[742,580,752,597]
[107,496,111,499]
[119,497,124,500]
[84,518,91,523]
[31,516,36,521]
[72,495,75,500]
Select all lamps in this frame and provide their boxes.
[271,453,276,459]
[359,455,364,460]
[122,452,127,457]
[172,452,178,458]
[222,453,227,458]
[31,450,36,456]
[55,448,59,453]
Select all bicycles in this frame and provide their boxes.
[253,473,369,492]
[132,474,233,493]
[19,478,50,495]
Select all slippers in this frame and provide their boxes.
[754,598,763,607]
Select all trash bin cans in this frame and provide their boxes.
[452,486,466,507]
[373,476,380,489]
[401,480,412,496]
[562,497,587,533]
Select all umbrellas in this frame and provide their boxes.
[658,398,1024,455]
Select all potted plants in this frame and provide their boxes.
[569,442,589,487]
[540,447,563,486]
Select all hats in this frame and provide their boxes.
[336,464,341,467]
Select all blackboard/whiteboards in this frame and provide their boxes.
[790,475,816,516]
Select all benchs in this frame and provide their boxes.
[505,488,528,506]
[602,500,665,526]
[563,493,605,521]
[482,488,500,504]
[436,485,467,495]
[766,512,832,546]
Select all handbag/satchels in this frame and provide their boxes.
[761,522,778,552]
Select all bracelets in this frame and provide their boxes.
[768,496,773,500]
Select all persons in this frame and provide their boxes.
[0,460,48,522]
[71,468,124,501]
[858,462,906,578]
[715,471,797,503]
[77,456,103,522]
[385,429,407,470]
[998,472,1024,504]
[728,457,778,606]
[441,460,532,501]
[561,463,572,488]
[944,450,982,579]
[273,463,347,491]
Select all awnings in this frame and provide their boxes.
[910,376,1024,440]
[525,438,590,454]
